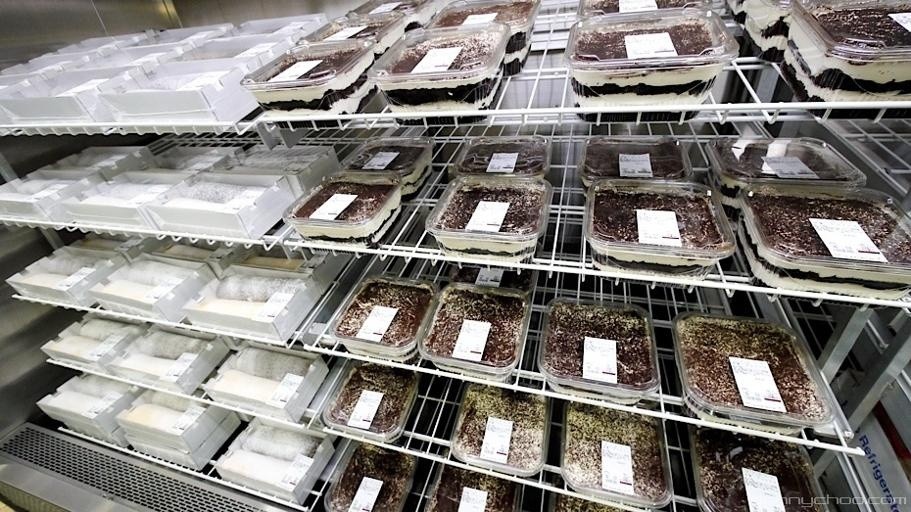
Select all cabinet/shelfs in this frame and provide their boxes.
[0,0,911,512]
